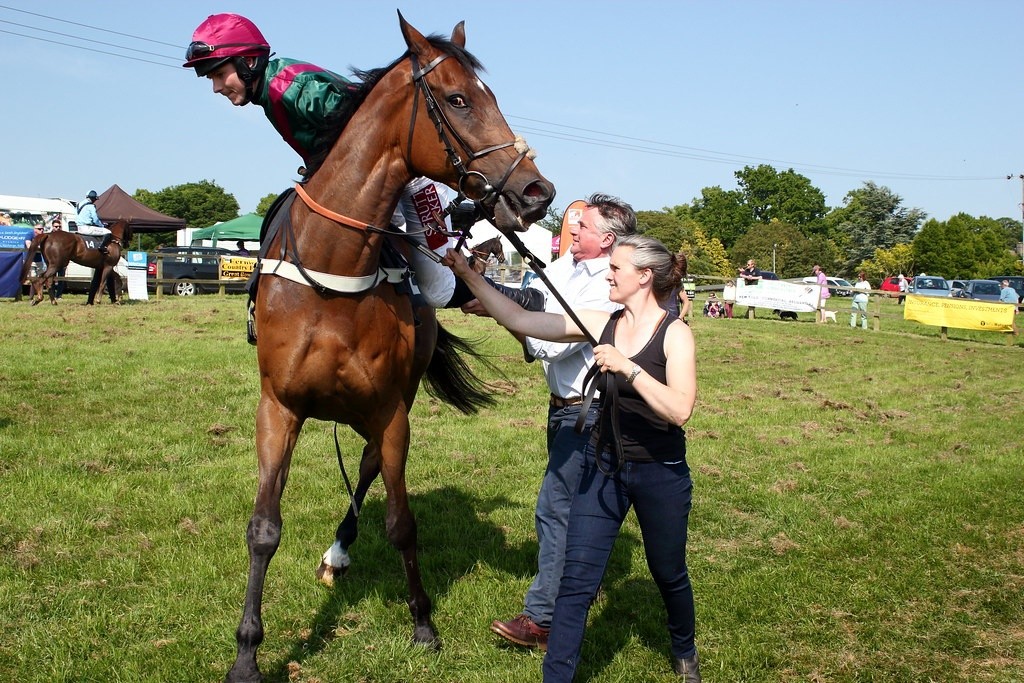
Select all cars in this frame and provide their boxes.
[734,270,781,285]
[906,273,954,299]
[961,280,1003,302]
[987,276,1024,303]
[944,279,968,297]
[825,280,850,297]
[879,278,914,297]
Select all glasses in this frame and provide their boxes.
[53,226,62,229]
[185,42,212,61]
[35,228,44,231]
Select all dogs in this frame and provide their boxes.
[772,309,798,322]
[821,310,838,323]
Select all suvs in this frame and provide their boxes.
[146,245,254,296]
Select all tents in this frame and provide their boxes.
[191,213,264,247]
[93,184,186,251]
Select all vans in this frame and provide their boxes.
[802,275,853,295]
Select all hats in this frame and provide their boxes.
[88,190,99,200]
[183,14,270,68]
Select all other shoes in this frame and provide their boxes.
[674,653,701,683]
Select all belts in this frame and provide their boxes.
[549,394,600,407]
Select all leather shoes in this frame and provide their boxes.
[491,611,553,652]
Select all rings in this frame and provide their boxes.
[599,359,603,363]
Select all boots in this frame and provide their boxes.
[445,273,547,364]
[450,203,480,231]
[98,234,112,255]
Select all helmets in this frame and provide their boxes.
[194,56,230,76]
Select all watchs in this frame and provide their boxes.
[626,364,641,383]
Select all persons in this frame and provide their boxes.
[999,280,1020,335]
[75,190,113,254]
[24,220,65,300]
[851,273,871,329]
[183,13,549,363]
[740,260,762,318]
[812,265,830,321]
[898,274,908,304]
[725,280,735,318]
[658,281,689,321]
[703,293,724,318]
[461,193,638,651]
[441,235,702,683]
[158,240,167,248]
[237,241,250,254]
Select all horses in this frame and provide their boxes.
[17,214,133,308]
[227,8,555,683]
